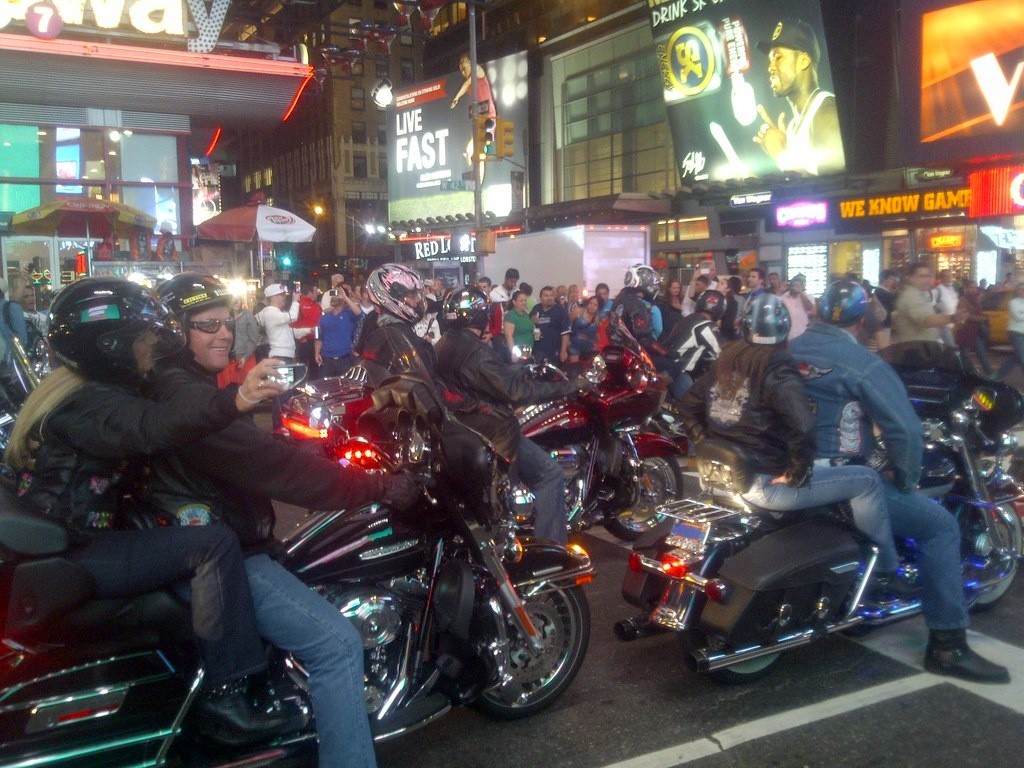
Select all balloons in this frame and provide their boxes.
[310,0,442,86]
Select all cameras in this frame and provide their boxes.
[330,290,338,296]
[263,363,307,388]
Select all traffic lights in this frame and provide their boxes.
[495,118,514,160]
[477,117,494,162]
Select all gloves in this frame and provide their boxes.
[562,379,591,395]
[379,472,430,512]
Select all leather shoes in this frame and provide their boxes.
[923,642,1011,683]
[866,572,925,599]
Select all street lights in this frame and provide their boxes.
[314,206,356,285]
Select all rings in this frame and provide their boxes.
[257,380,264,388]
[760,123,770,133]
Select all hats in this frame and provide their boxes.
[0,277,8,294]
[264,284,285,297]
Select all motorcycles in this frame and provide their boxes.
[278,321,688,546]
[0,323,597,767]
[613,340,1023,688]
[0,322,56,451]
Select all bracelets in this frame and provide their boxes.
[239,386,264,403]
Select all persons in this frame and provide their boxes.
[150,274,423,768]
[433,285,593,546]
[608,262,726,474]
[665,261,817,342]
[289,274,375,387]
[0,275,288,743]
[230,297,260,370]
[675,294,927,600]
[256,283,302,437]
[784,279,1010,684]
[356,262,517,521]
[476,268,615,379]
[451,55,496,186]
[833,264,1024,394]
[753,17,845,171]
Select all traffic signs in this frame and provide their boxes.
[468,99,490,119]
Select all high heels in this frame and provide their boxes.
[191,673,290,748]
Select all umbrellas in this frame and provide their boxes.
[8,197,316,284]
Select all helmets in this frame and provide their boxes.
[625,263,662,288]
[820,279,869,327]
[47,276,168,369]
[442,285,489,328]
[366,263,426,323]
[156,272,232,314]
[696,290,727,321]
[743,294,792,345]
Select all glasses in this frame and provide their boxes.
[188,317,236,334]
